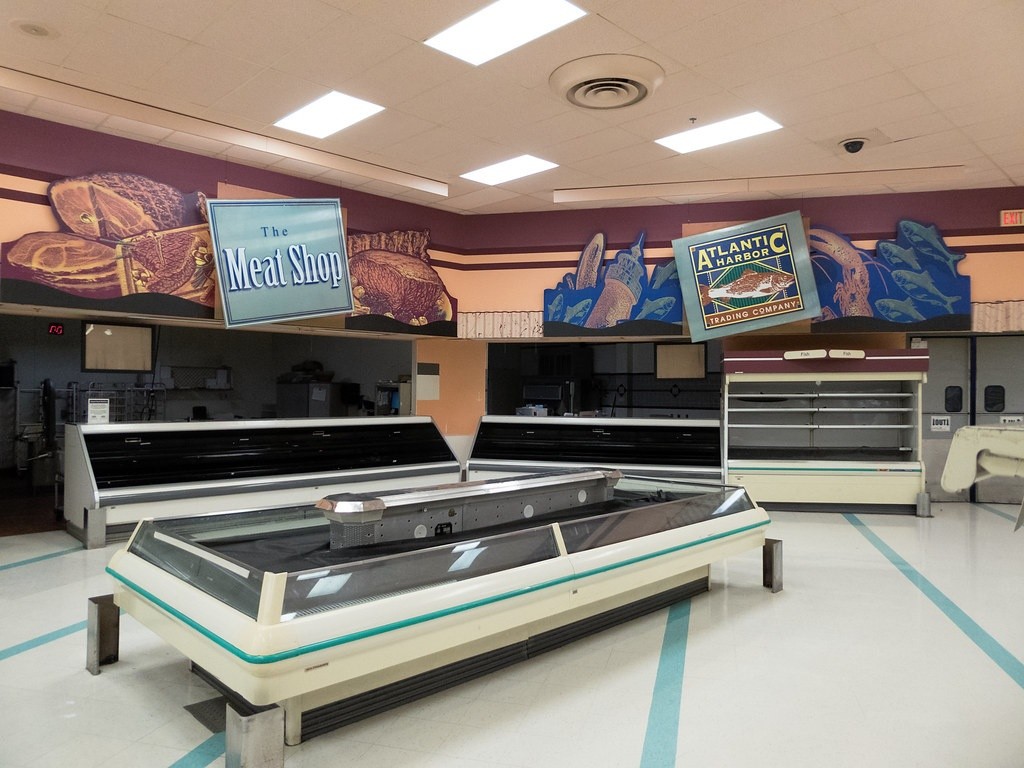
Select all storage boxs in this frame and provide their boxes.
[516,408,546,417]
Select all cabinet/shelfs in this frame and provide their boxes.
[720,347,930,516]
